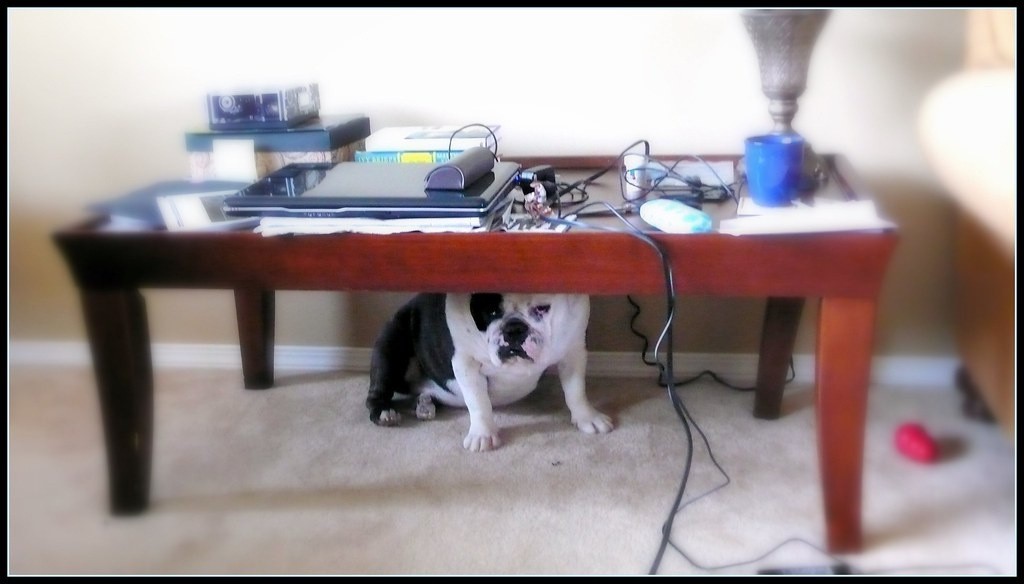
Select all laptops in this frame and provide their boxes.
[222,160,520,221]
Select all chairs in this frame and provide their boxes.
[917,8,1014,444]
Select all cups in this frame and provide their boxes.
[742,133,804,209]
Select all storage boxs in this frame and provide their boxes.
[185,115,372,183]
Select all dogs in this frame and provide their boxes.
[365,292,615,453]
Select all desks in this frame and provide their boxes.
[50,151,899,555]
